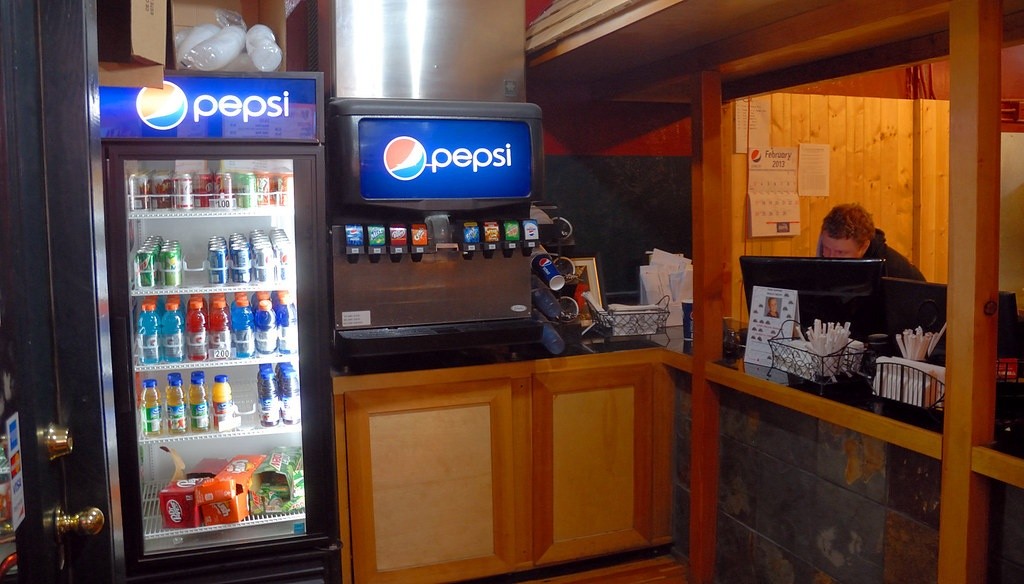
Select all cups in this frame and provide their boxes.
[175,23,283,72]
[681,299,694,340]
[542,324,565,355]
[532,254,564,290]
[534,289,561,317]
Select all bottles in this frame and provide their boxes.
[213,374,234,430]
[137,290,297,365]
[257,363,301,427]
[140,380,164,436]
[165,371,210,434]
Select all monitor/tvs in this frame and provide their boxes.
[736,255,1020,360]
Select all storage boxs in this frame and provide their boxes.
[166,0,287,74]
[158,445,304,528]
[95,0,177,89]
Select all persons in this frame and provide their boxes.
[767,298,778,318]
[816,205,927,282]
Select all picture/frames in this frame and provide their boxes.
[571,257,603,325]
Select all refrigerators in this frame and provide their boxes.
[97,71,342,584]
